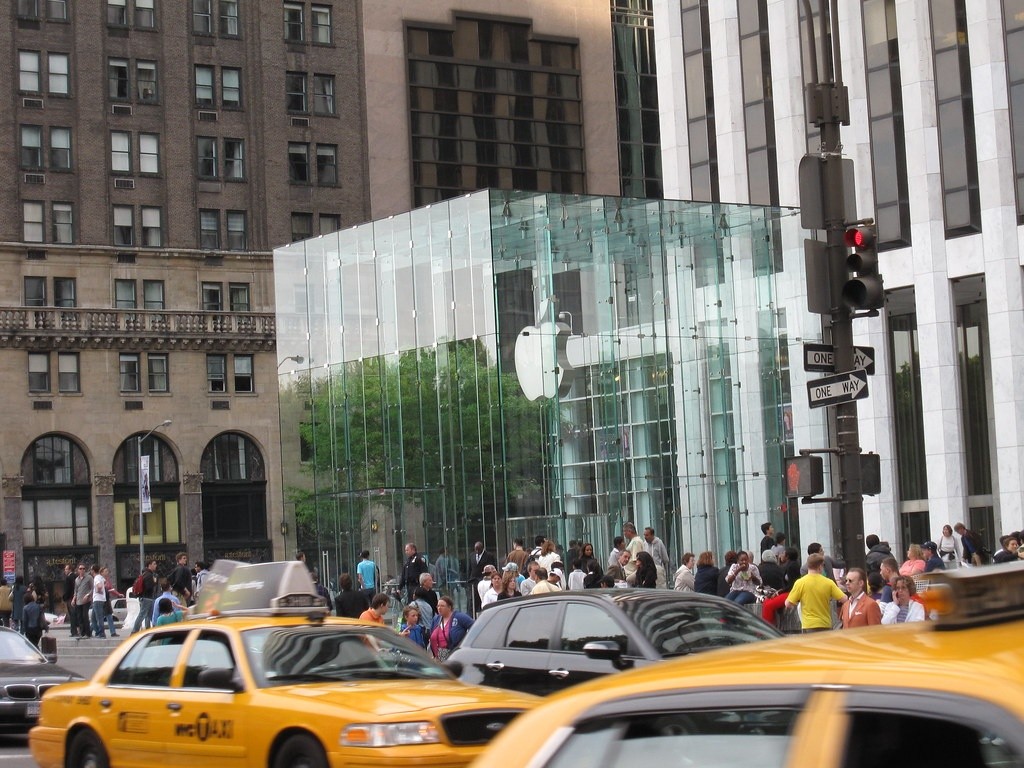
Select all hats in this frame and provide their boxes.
[921,541,937,550]
[482,565,496,573]
[549,568,563,582]
[502,562,519,571]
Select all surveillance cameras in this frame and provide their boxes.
[559,313,566,320]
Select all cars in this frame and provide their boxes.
[446,587,784,696]
[44,613,59,625]
[463,561,1024,768]
[0,626,87,734]
[28,561,548,768]
[88,598,128,622]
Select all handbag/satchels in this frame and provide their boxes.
[521,555,535,579]
[437,648,450,662]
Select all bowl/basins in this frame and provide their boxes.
[741,572,751,580]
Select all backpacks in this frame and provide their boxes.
[133,571,148,596]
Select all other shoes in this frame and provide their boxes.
[111,633,120,637]
[69,633,79,637]
[99,635,106,639]
[76,635,90,639]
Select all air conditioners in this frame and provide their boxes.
[23,174,46,184]
[197,111,219,121]
[111,104,134,117]
[113,177,135,190]
[290,116,310,127]
[22,98,44,109]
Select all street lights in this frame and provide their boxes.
[138,420,172,575]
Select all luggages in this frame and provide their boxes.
[41,632,57,663]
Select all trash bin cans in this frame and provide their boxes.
[40,637,58,663]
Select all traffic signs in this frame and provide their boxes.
[807,369,870,409]
[804,343,875,375]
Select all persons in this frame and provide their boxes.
[555,545,564,564]
[131,552,209,635]
[467,522,670,621]
[0,575,49,652]
[673,522,1024,635]
[63,565,124,640]
[295,552,333,616]
[434,546,452,597]
[336,542,474,668]
[567,540,580,590]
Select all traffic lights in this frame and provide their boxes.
[785,455,824,498]
[844,224,885,311]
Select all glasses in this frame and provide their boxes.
[845,579,859,583]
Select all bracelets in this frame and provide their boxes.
[733,573,736,577]
